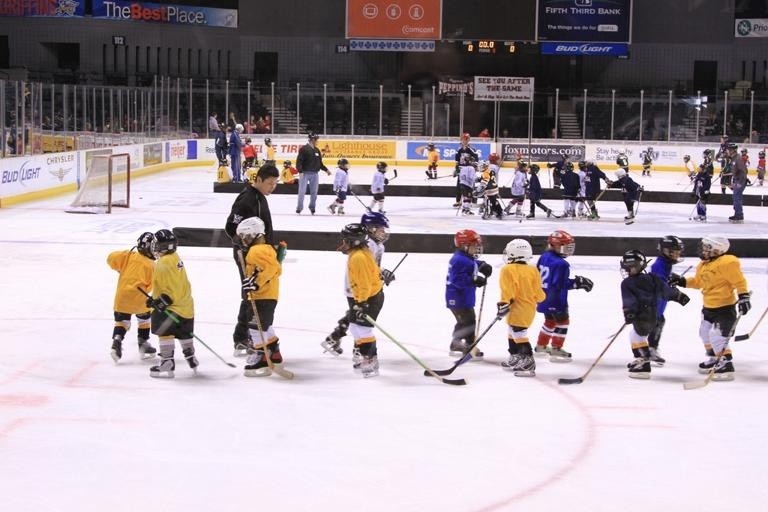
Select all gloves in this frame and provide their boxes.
[668,272,686,286]
[241,271,259,297]
[479,262,492,277]
[575,275,594,293]
[352,302,370,320]
[474,276,487,288]
[671,290,690,306]
[497,301,511,320]
[380,270,397,286]
[737,292,753,316]
[146,293,174,314]
[623,306,641,325]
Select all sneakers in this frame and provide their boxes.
[295,206,304,213]
[450,340,468,352]
[337,205,345,213]
[552,346,572,358]
[234,339,253,351]
[361,354,381,374]
[353,348,362,365]
[534,345,551,354]
[456,208,746,220]
[309,206,316,213]
[330,202,336,214]
[650,347,665,362]
[464,346,484,356]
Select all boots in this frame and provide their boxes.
[699,350,715,368]
[112,339,124,359]
[627,355,651,373]
[150,351,176,373]
[181,347,199,367]
[710,353,734,375]
[501,351,520,368]
[267,337,282,364]
[137,339,156,353]
[244,344,271,368]
[512,353,536,372]
[326,326,347,354]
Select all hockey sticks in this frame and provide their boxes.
[683,289,753,390]
[689,200,700,221]
[578,191,600,221]
[735,307,768,341]
[519,194,527,222]
[608,266,693,337]
[424,315,502,377]
[551,213,562,218]
[425,170,454,178]
[746,176,757,186]
[558,321,627,385]
[352,304,466,384]
[237,248,294,379]
[454,276,488,365]
[368,169,397,191]
[498,194,515,216]
[625,185,645,225]
[135,285,240,368]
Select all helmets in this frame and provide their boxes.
[701,232,731,260]
[308,133,319,142]
[216,121,292,167]
[150,229,178,256]
[338,158,349,169]
[341,222,370,242]
[619,249,648,278]
[452,133,631,172]
[376,162,387,172]
[137,232,154,252]
[360,212,390,244]
[656,235,684,263]
[236,216,266,239]
[548,230,575,257]
[504,238,533,261]
[453,229,483,259]
[648,134,768,163]
[614,168,627,177]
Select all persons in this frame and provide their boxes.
[236,217,283,368]
[619,249,669,372]
[145,229,199,372]
[223,163,278,351]
[444,230,492,357]
[649,236,684,362]
[496,238,546,372]
[8,102,197,154]
[107,231,157,359]
[537,230,593,357]
[325,212,395,355]
[667,231,751,374]
[337,224,384,373]
